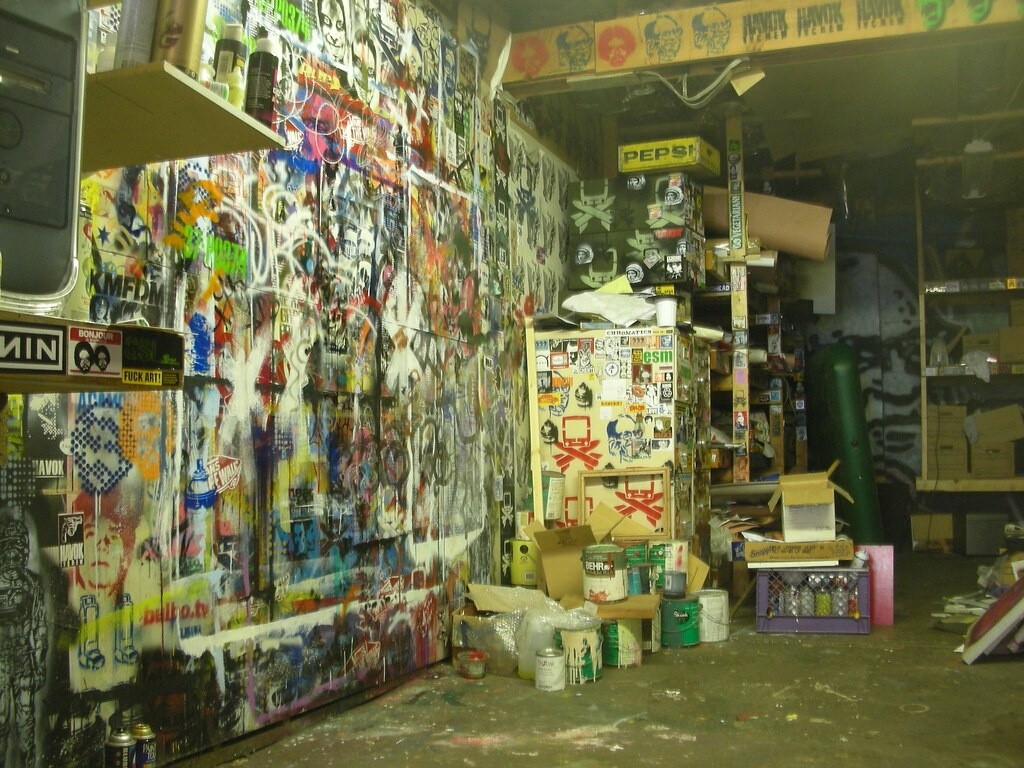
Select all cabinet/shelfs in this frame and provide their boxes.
[691,114,809,485]
[910,106,1024,526]
[523,316,714,592]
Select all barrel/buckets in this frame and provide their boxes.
[554,623,603,682]
[642,609,661,654]
[663,571,686,599]
[648,538,689,587]
[660,593,701,647]
[613,539,648,567]
[515,511,536,541]
[581,542,628,602]
[602,618,642,667]
[524,470,565,520]
[509,537,540,585]
[626,562,656,596]
[535,647,565,692]
[691,589,730,643]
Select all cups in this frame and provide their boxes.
[656,298,678,327]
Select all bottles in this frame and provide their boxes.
[815,585,831,617]
[213,23,247,111]
[245,38,281,131]
[131,724,157,768]
[784,585,799,616]
[849,548,869,569]
[800,585,815,616]
[105,726,136,768]
[831,586,849,618]
[848,586,858,617]
[152,0,207,83]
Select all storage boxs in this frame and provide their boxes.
[725,459,873,635]
[557,134,720,325]
[450,602,518,675]
[523,501,625,600]
[958,507,1009,555]
[1004,205,1023,279]
[909,508,957,554]
[926,403,1024,480]
[962,300,1024,364]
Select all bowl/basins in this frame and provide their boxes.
[457,651,490,680]
